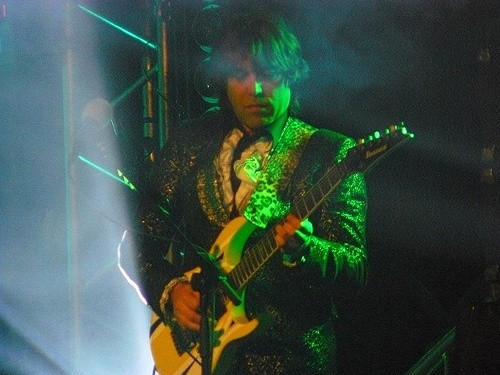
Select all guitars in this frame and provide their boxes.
[150,122,414,375]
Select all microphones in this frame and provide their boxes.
[67,99,113,171]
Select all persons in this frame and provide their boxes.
[130,12,370,375]
